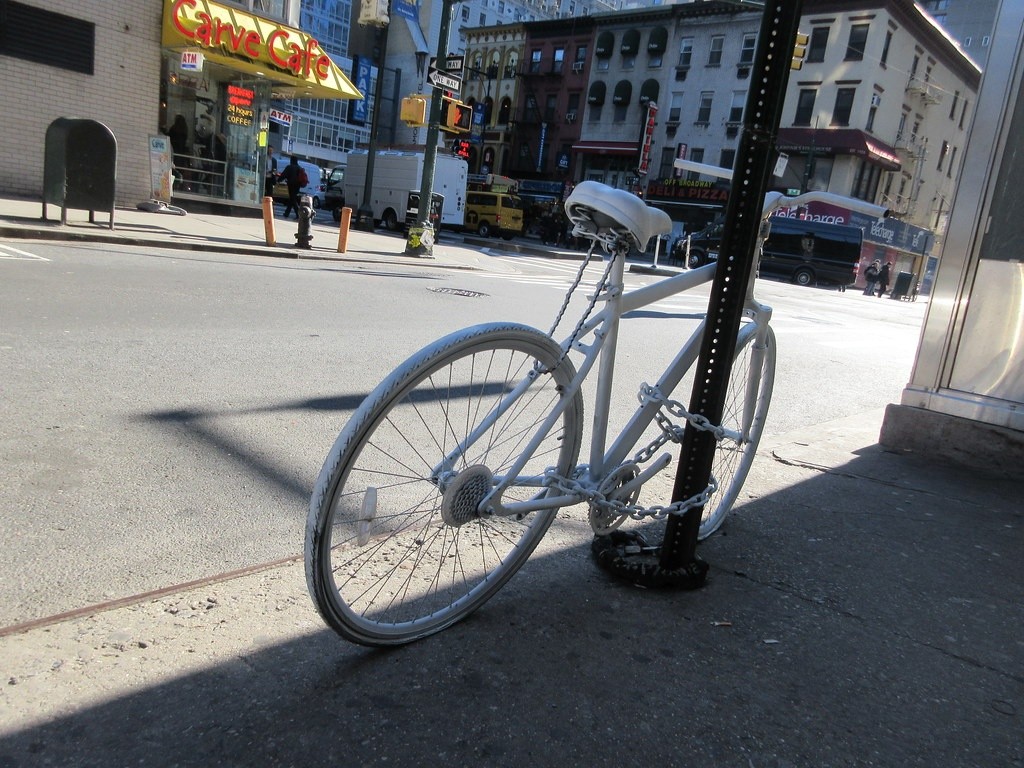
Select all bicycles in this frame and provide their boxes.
[302,156,900,649]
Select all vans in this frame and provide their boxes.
[673,213,865,290]
[459,190,524,241]
[273,159,327,209]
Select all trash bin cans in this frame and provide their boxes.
[403,190,444,244]
[44,116,118,213]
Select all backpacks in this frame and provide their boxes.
[298,168,308,187]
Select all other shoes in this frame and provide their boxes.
[283,212,289,219]
[293,214,299,220]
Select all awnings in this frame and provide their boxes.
[774,124,903,174]
[572,139,640,155]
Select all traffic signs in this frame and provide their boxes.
[428,55,467,72]
[427,66,462,95]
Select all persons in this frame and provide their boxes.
[863,262,879,296]
[277,156,304,220]
[517,196,584,252]
[266,145,277,197]
[864,259,881,297]
[877,262,892,298]
[162,114,188,191]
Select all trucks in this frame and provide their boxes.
[322,152,469,233]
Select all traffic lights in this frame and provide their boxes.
[440,98,475,134]
